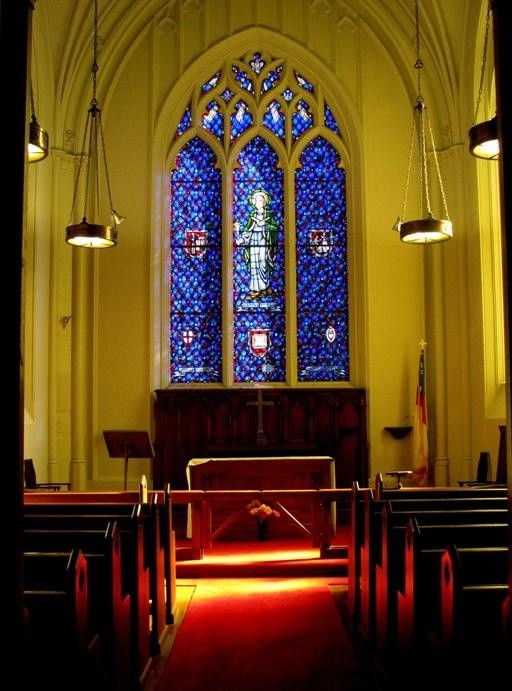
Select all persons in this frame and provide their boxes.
[234,190,279,300]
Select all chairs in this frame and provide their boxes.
[456,453,492,487]
[25,458,72,492]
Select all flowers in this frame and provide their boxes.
[245,498,281,522]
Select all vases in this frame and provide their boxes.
[255,516,269,541]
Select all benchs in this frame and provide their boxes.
[21,483,184,691]
[342,471,512,691]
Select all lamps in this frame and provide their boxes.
[25,2,124,249]
[389,2,499,246]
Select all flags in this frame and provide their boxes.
[412,349,428,487]
